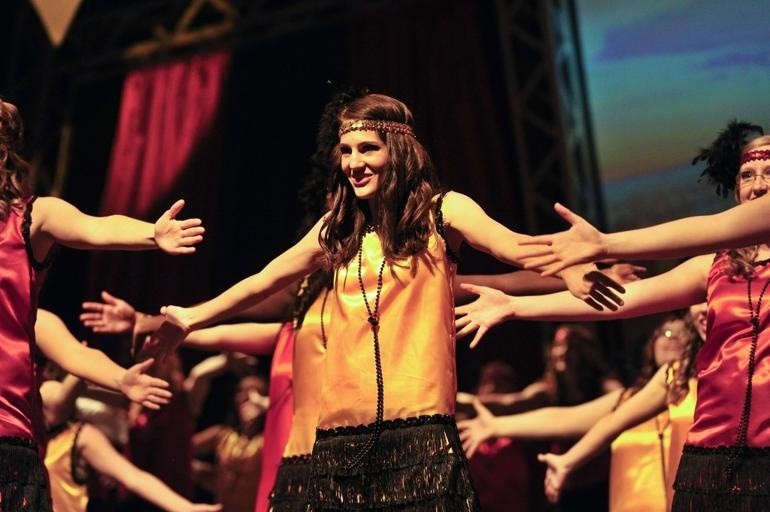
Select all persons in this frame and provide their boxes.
[453,263,713,512]
[0,101,204,512]
[455,134,770,511]
[145,93,624,511]
[515,189,770,277]
[24,269,335,512]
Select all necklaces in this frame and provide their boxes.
[723,241,770,479]
[344,219,389,473]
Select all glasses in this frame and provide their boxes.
[733,169,770,185]
